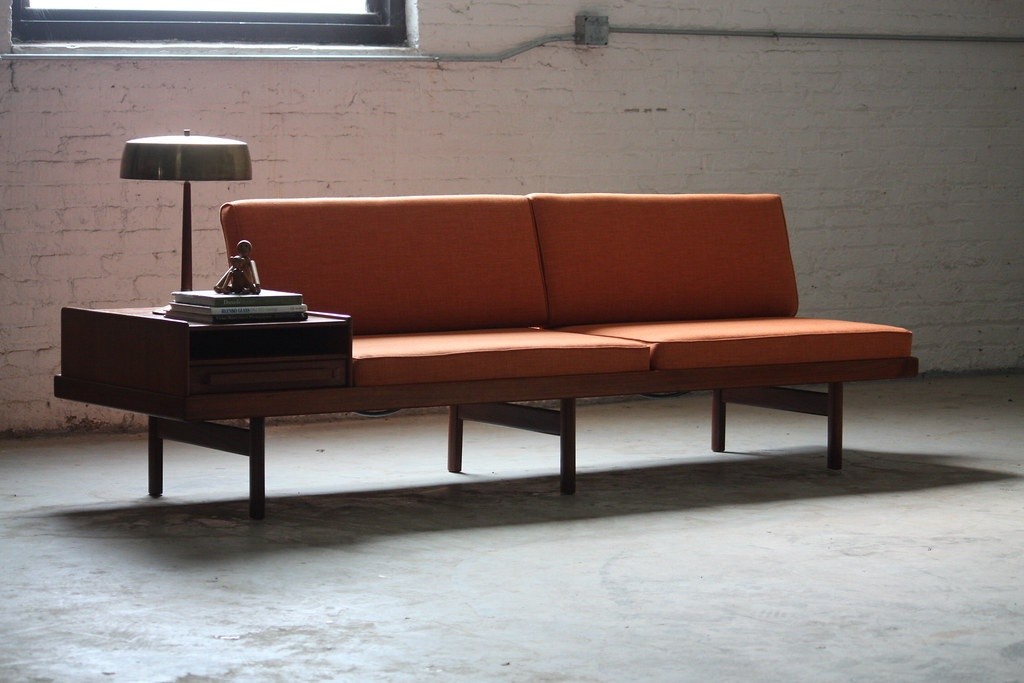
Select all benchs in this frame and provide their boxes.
[219,194,920,497]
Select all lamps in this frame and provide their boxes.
[118,128,254,316]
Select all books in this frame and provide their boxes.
[164,288,308,323]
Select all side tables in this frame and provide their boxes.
[55,307,353,519]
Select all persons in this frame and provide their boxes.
[213,240,261,295]
